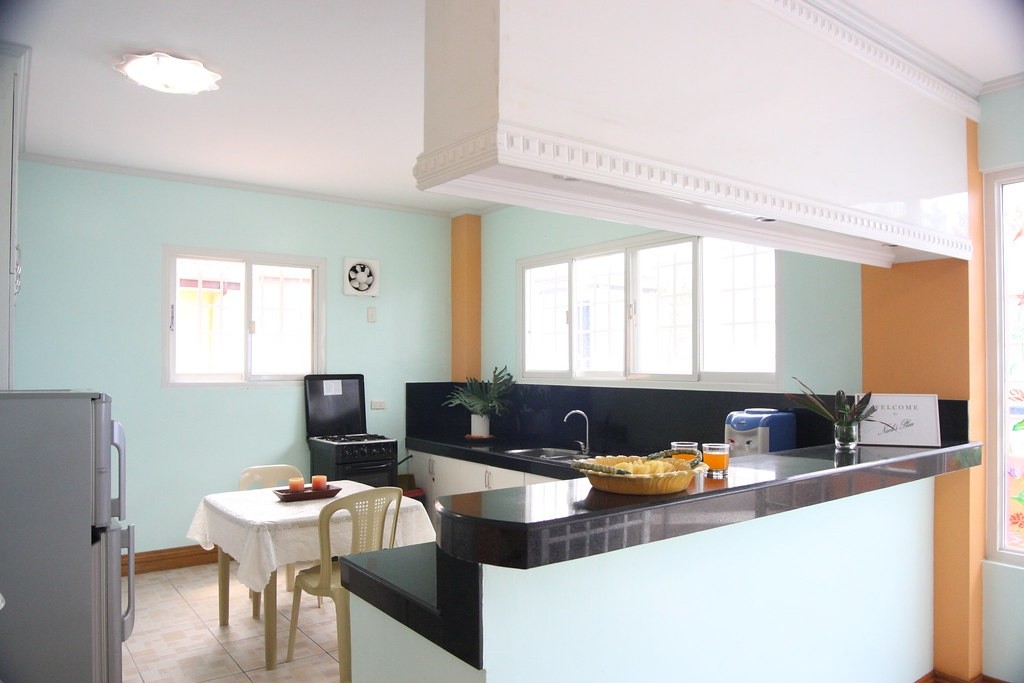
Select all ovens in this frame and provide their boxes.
[310,460,398,487]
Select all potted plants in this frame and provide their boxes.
[787,376,878,450]
[439,364,518,443]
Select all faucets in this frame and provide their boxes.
[745,440,752,451]
[563,408,590,455]
[728,439,735,451]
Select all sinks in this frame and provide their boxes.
[504,447,581,460]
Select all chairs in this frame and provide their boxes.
[239,464,404,683]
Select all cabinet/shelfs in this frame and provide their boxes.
[407,449,566,525]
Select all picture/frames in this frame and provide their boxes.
[853,393,941,447]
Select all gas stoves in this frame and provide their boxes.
[309,434,398,464]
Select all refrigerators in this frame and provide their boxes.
[0,389,135,683]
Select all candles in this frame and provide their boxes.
[312,475,328,491]
[289,477,305,492]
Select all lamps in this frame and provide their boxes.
[112,52,221,95]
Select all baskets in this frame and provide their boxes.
[572,447,709,494]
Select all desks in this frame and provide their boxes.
[186,480,437,672]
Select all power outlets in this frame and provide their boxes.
[371,400,385,410]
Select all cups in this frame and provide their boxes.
[702,443,730,480]
[671,442,698,460]
